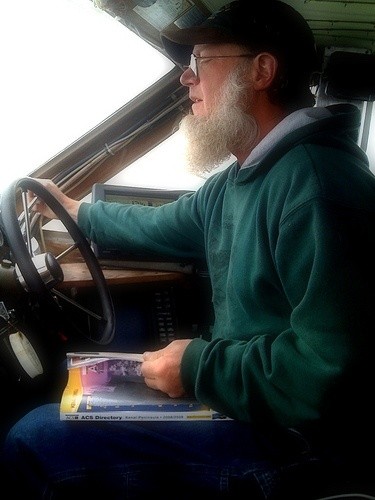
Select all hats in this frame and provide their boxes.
[162,1,320,73]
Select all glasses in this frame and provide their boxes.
[188,53,254,77]
